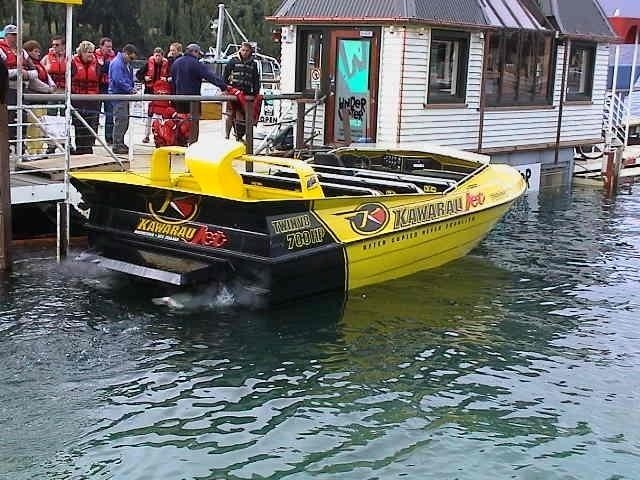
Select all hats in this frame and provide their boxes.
[186,44,205,55]
[4,25,17,34]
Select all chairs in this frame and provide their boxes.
[315,152,345,167]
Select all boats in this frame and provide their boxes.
[66,138,527,310]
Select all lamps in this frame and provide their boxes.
[389,24,395,33]
[554,30,560,39]
[288,24,294,32]
[480,29,485,39]
[418,24,425,35]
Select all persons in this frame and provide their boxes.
[1,21,234,155]
[218,41,261,141]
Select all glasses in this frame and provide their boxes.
[52,43,59,46]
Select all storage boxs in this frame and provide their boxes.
[199,100,222,120]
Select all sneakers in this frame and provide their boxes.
[143,137,149,143]
[16,138,128,161]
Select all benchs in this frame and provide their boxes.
[241,163,458,198]
